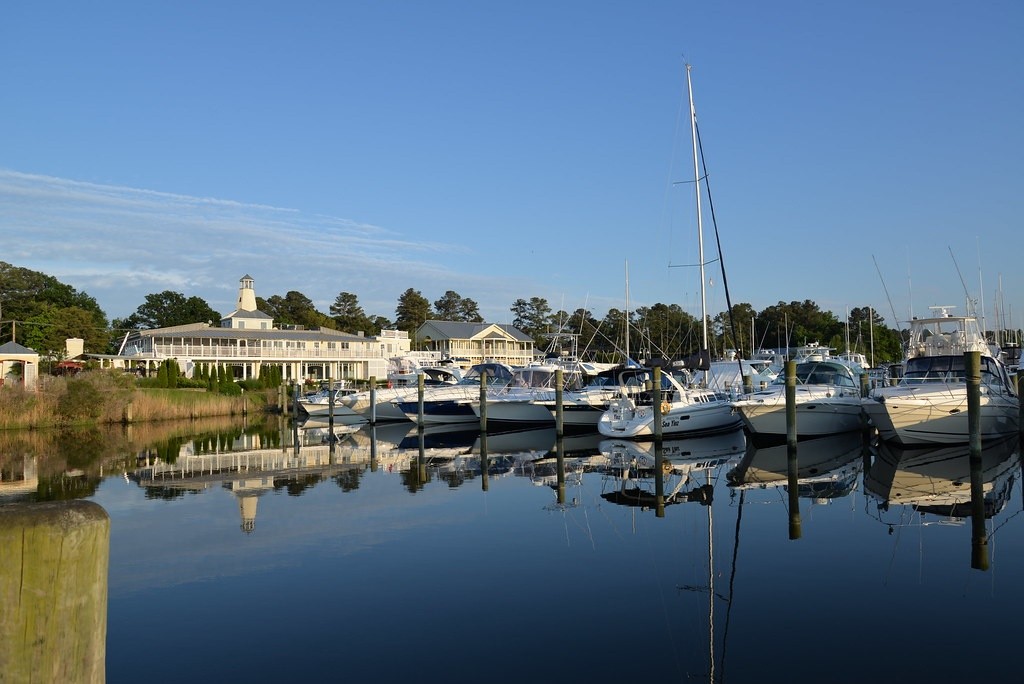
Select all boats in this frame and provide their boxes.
[863,433,1024,535]
[300,414,747,684]
[299,67,1022,444]
[724,426,880,507]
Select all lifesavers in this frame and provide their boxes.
[662,460,672,475]
[660,401,671,414]
